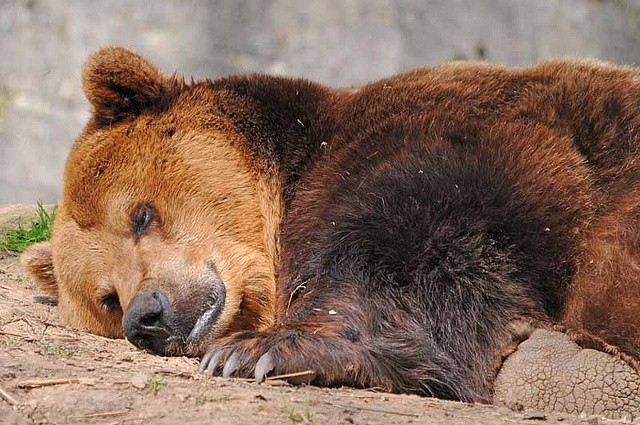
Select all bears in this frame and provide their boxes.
[18,46,640,425]
